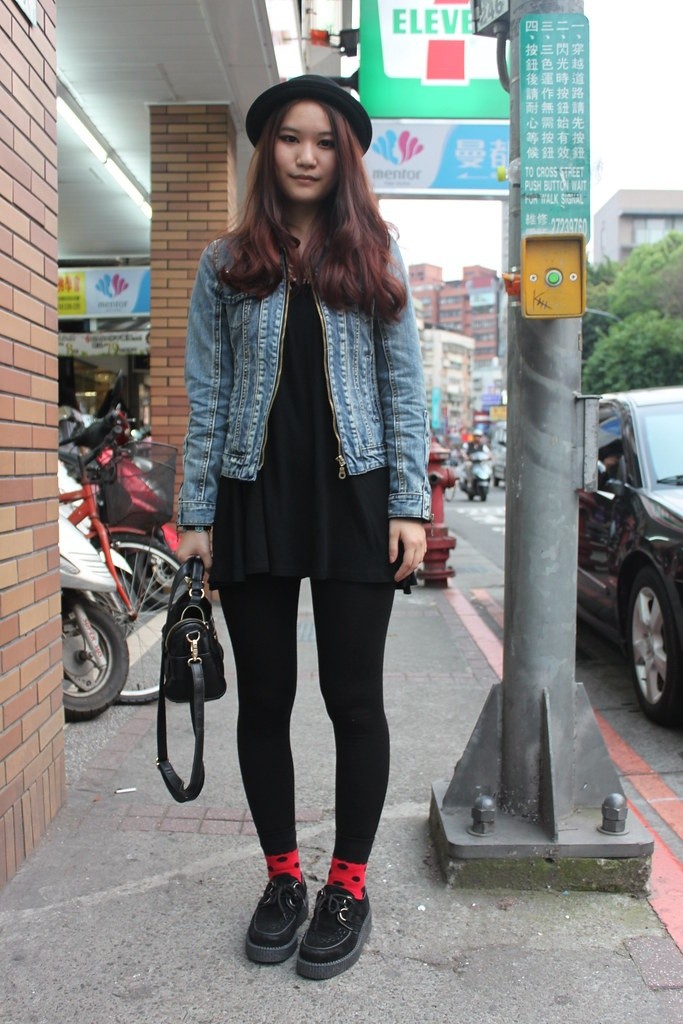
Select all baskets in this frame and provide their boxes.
[96,440,179,530]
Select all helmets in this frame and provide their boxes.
[472,429,483,436]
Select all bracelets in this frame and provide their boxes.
[183,526,211,532]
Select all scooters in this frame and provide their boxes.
[58,369,180,604]
[460,452,491,501]
[58,512,131,723]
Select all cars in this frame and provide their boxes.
[579,386,682,727]
[490,422,507,487]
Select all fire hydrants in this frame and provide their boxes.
[417,437,456,588]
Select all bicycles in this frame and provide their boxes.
[59,407,182,708]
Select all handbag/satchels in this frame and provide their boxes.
[162,557,228,703]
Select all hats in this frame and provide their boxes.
[244,75,373,158]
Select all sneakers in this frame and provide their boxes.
[245,871,309,963]
[297,885,371,980]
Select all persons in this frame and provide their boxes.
[176,74,427,975]
[463,430,492,488]
